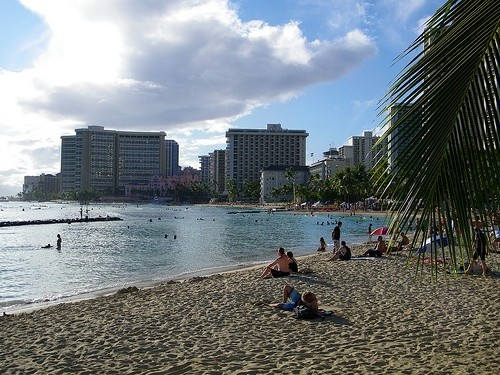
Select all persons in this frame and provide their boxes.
[465,227,490,277]
[408,214,457,238]
[57,234,61,250]
[260,247,291,279]
[287,251,300,272]
[367,223,373,234]
[318,237,327,251]
[329,242,351,261]
[400,232,410,245]
[332,222,343,253]
[488,220,500,252]
[268,285,319,314]
[357,235,385,257]
